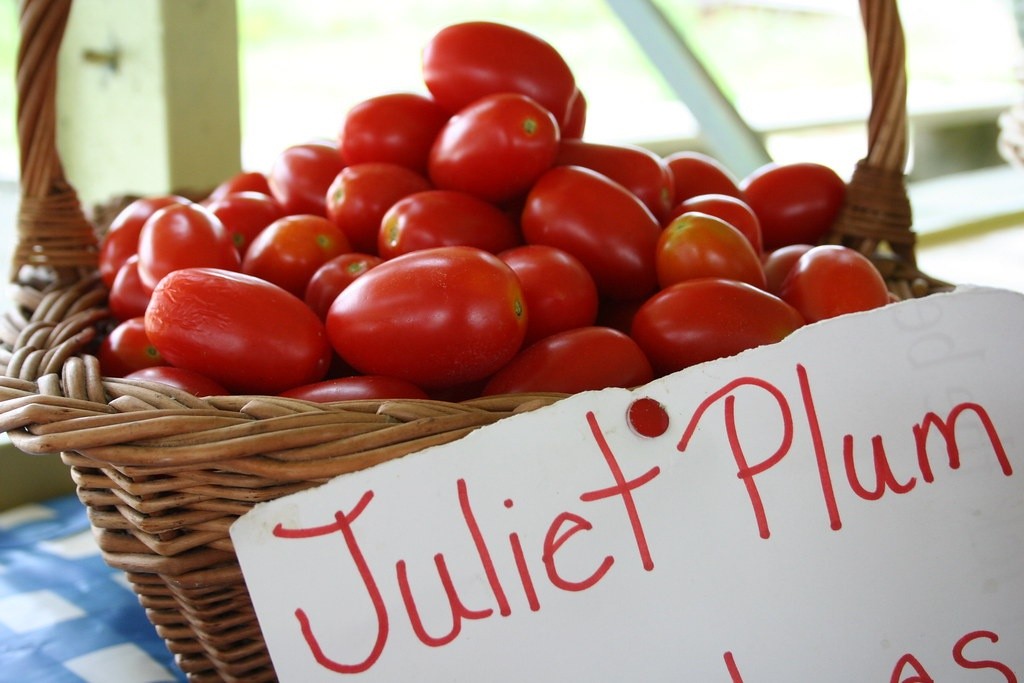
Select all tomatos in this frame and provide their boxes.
[96,21,903,401]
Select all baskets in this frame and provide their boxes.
[2,0,950,683]
